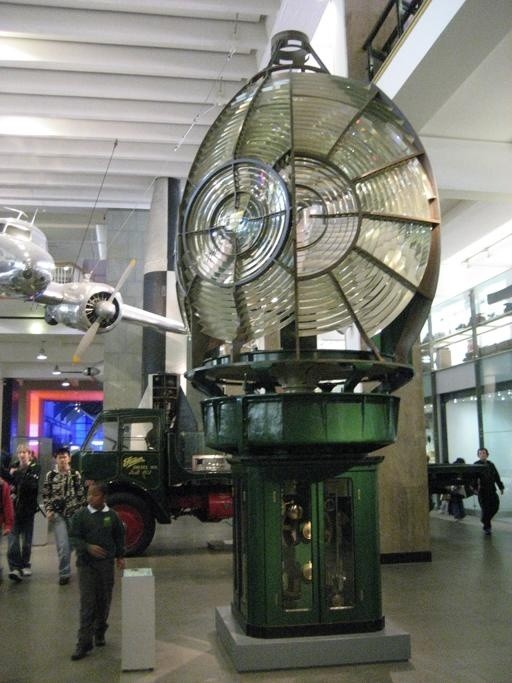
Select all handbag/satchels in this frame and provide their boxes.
[17,478,37,501]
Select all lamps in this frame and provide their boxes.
[61,378,71,387]
[51,365,62,375]
[36,342,48,360]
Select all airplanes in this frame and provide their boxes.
[0,197,188,369]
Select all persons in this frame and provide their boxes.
[41,449,86,586]
[67,483,125,659]
[426,455,467,521]
[474,447,504,535]
[6,445,40,581]
[0,476,14,586]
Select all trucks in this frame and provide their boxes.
[61,398,492,564]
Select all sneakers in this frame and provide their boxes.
[7,567,33,582]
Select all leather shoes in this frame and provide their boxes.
[95,634,106,645]
[59,576,70,585]
[72,643,94,660]
